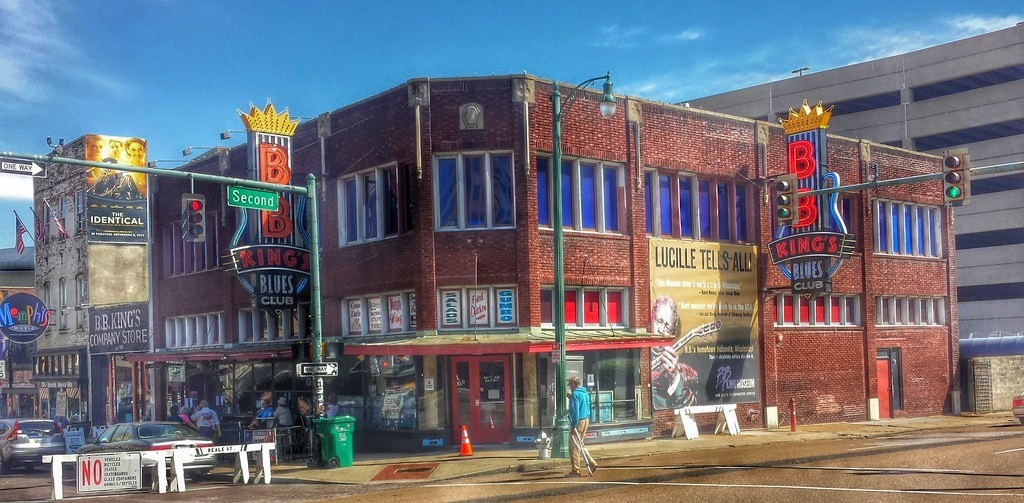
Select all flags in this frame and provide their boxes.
[15,218,26,253]
[48,205,69,236]
[34,216,46,241]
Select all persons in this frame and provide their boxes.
[250,395,339,429]
[86,135,146,199]
[169,400,222,441]
[566,376,597,476]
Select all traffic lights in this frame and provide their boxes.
[181,192,207,243]
[941,147,972,202]
[772,170,801,227]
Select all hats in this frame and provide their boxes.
[567,375,581,383]
[170,405,178,413]
[277,397,287,405]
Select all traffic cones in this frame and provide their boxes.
[461,424,473,457]
[488,415,494,430]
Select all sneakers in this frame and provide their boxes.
[565,471,581,477]
[589,461,597,477]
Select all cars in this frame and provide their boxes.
[74,420,216,480]
[0,417,68,473]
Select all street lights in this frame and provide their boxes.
[546,70,618,453]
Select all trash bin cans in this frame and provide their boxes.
[312,416,356,469]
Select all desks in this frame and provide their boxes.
[223,413,255,442]
[257,416,280,429]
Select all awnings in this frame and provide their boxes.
[344,330,675,355]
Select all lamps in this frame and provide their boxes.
[182,146,212,157]
[148,158,189,169]
[219,129,247,141]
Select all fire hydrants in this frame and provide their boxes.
[789,398,796,430]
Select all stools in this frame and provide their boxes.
[219,421,306,464]
